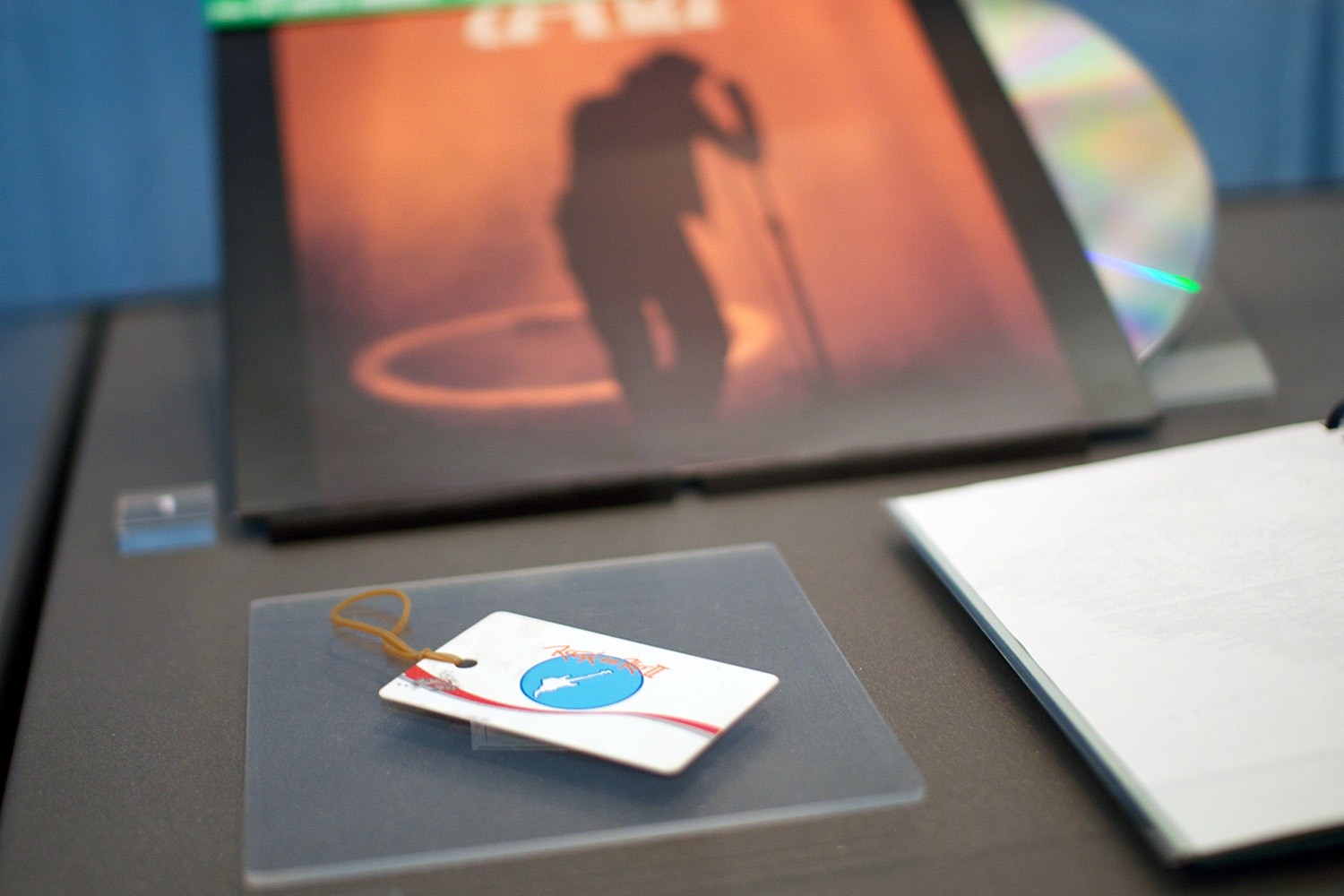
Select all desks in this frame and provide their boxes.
[0,193,1343,896]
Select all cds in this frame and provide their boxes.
[963,0,1214,364]
